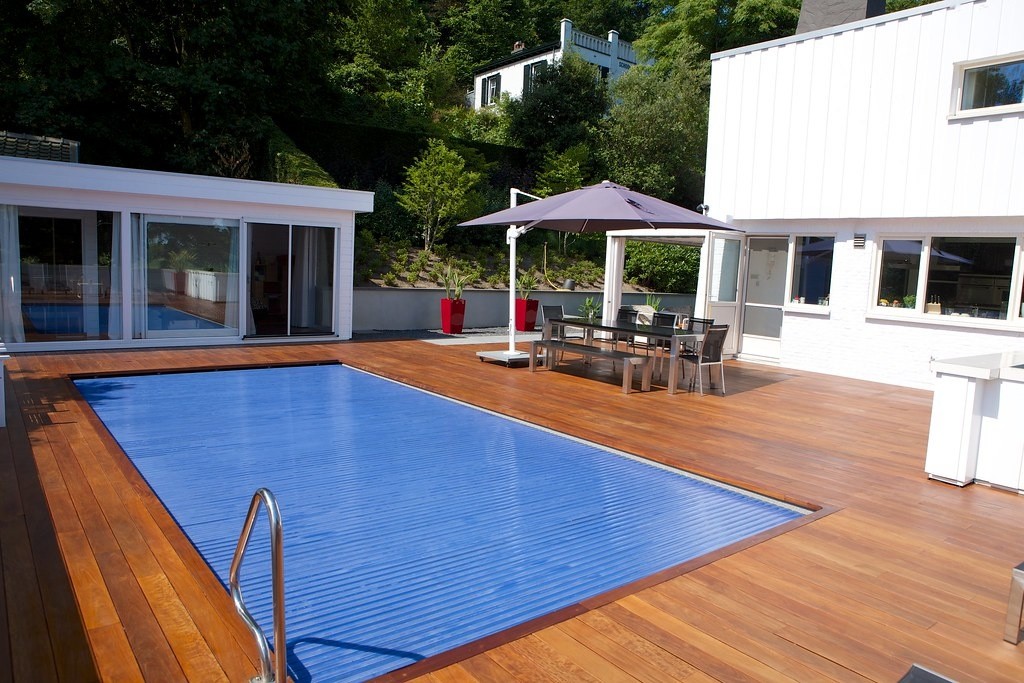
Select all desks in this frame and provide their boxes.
[75,282,105,299]
[547,311,717,395]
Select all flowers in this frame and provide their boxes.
[168,247,194,272]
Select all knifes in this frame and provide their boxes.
[931,294,940,304]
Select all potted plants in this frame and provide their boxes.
[161,262,239,302]
[429,264,476,335]
[515,264,540,332]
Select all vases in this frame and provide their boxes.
[170,270,186,298]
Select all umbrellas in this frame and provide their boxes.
[796,236,973,301]
[456,178,747,353]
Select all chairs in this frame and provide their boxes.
[628,309,679,379]
[679,322,733,398]
[539,304,583,362]
[660,317,715,384]
[588,306,640,372]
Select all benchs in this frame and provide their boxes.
[525,340,653,395]
